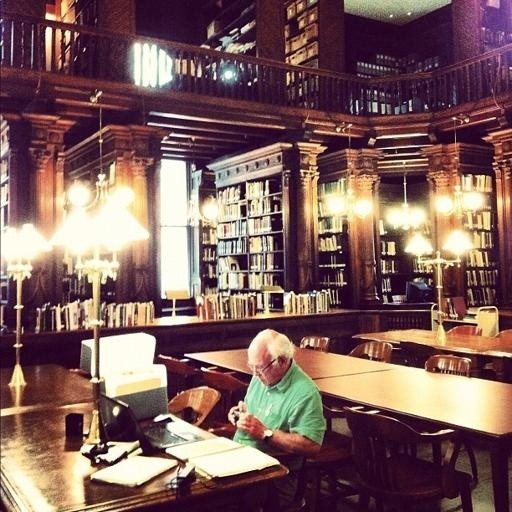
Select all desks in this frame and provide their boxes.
[2,361,293,511]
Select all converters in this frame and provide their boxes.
[176,463,197,487]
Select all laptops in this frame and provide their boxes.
[98,390,200,455]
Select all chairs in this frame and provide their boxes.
[70,325,512,511]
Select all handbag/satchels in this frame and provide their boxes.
[475,305,500,336]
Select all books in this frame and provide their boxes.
[1,156,497,333]
[80,414,280,488]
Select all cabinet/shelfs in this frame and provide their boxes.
[189,169,220,309]
[312,143,386,310]
[207,140,328,313]
[417,141,499,306]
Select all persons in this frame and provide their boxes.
[228,328,327,512]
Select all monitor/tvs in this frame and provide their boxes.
[405,281,432,303]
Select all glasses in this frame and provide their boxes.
[250,359,278,375]
